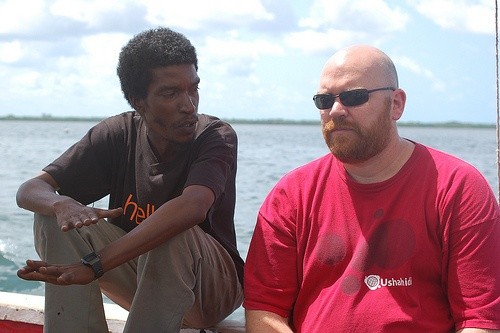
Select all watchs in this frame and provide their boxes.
[81,251,105,281]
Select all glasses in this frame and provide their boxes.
[312,86,397,110]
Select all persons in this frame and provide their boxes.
[243,42,499,333]
[15,27,245,332]
